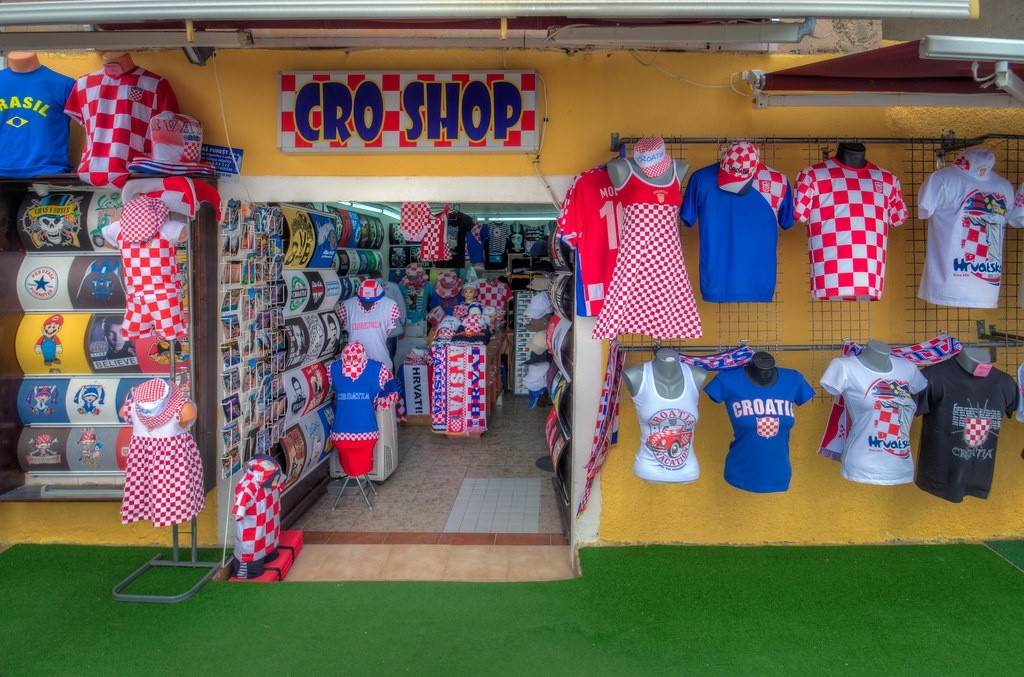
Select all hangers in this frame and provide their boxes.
[596,141,626,169]
[447,203,461,220]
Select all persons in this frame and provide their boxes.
[703,353,816,492]
[340,280,400,370]
[327,316,340,349]
[917,149,1015,308]
[64,52,177,184]
[292,322,307,355]
[793,142,909,301]
[820,340,928,486]
[398,262,514,322]
[102,194,188,340]
[914,346,1018,503]
[327,344,397,476]
[119,378,205,527]
[593,136,702,340]
[234,455,285,579]
[679,140,794,301]
[0,51,76,177]
[292,377,306,413]
[622,349,707,484]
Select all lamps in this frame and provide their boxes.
[181,27,216,65]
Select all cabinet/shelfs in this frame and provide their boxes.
[508,254,545,396]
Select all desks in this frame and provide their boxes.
[445,330,507,438]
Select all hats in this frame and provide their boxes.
[952,148,996,180]
[435,271,463,298]
[718,139,760,195]
[120,112,217,179]
[520,291,554,410]
[131,378,179,430]
[118,195,171,243]
[357,279,385,302]
[526,277,552,291]
[633,136,673,178]
[460,282,478,296]
[342,340,366,377]
[521,240,549,257]
[401,263,430,288]
[468,301,484,315]
